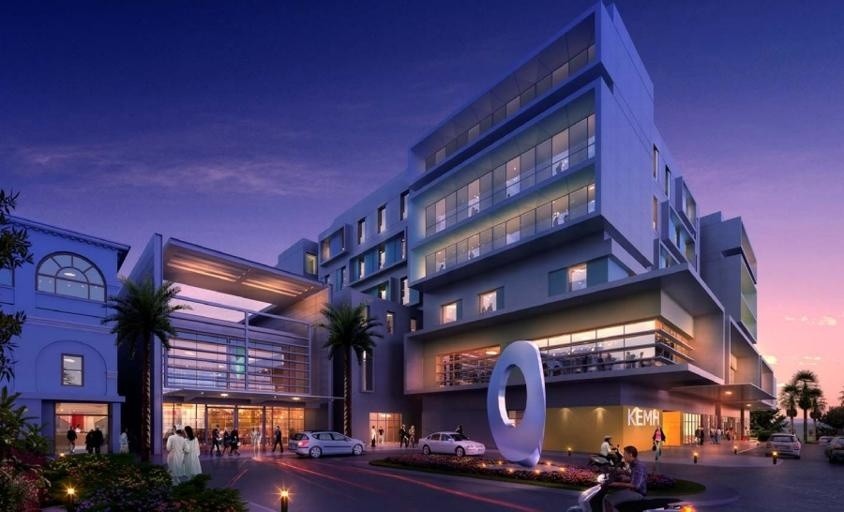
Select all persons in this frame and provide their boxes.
[272,425,284,453]
[455,424,464,434]
[66,425,78,452]
[118,426,129,454]
[439,264,444,272]
[409,425,416,448]
[399,424,409,448]
[552,217,559,226]
[652,426,666,461]
[165,424,184,485]
[694,426,736,448]
[74,424,81,433]
[370,426,376,447]
[377,426,384,445]
[85,429,94,454]
[184,426,203,483]
[469,251,475,260]
[556,162,562,174]
[453,342,674,384]
[563,214,568,223]
[603,445,648,512]
[94,428,104,454]
[600,435,617,468]
[210,423,262,458]
[471,206,478,216]
